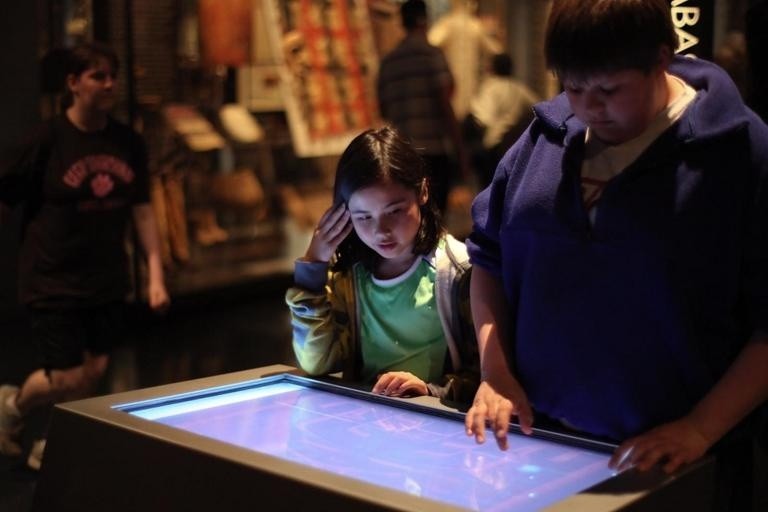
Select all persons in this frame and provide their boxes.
[373,2,748,217]
[283,126,467,406]
[460,0,768,512]
[1,40,170,473]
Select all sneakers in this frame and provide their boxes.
[26,438,48,472]
[0,383,30,460]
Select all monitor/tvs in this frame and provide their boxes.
[52,362,713,512]
